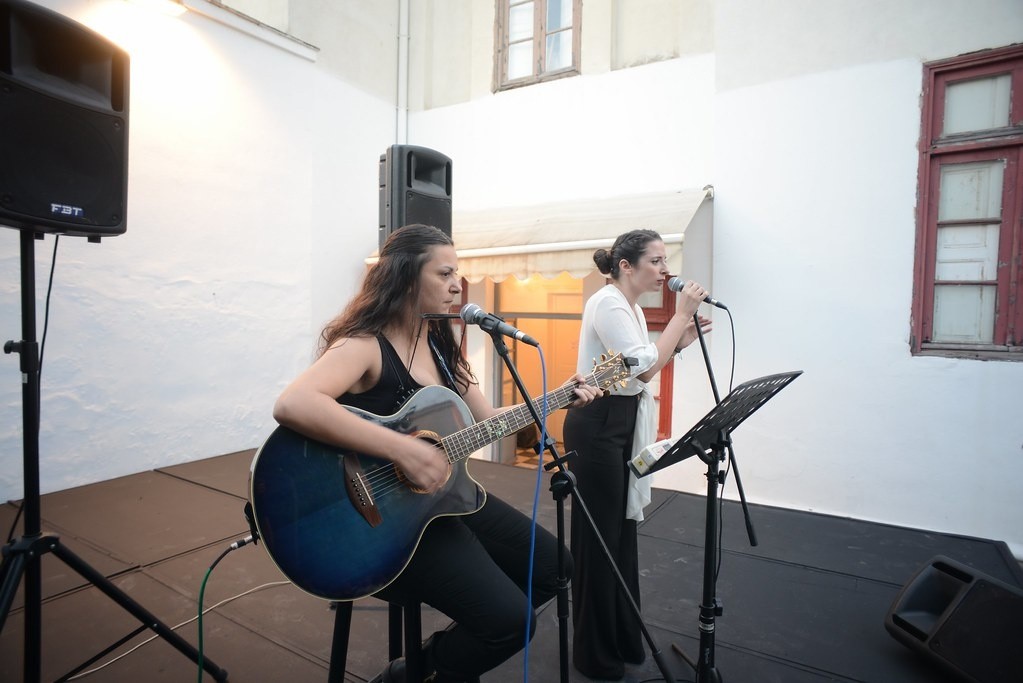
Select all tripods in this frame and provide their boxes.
[0,225,229,683]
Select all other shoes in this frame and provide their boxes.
[577,667,624,680]
[370,631,469,683]
[625,653,646,664]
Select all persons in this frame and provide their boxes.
[563,230,714,683]
[273,224,574,683]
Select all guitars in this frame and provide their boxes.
[248,352,634,602]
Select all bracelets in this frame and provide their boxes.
[674,347,682,353]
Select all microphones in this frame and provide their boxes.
[668,277,728,310]
[459,303,539,347]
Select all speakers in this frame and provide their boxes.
[0,0,131,243]
[379,144,453,257]
[883,555,1023,683]
